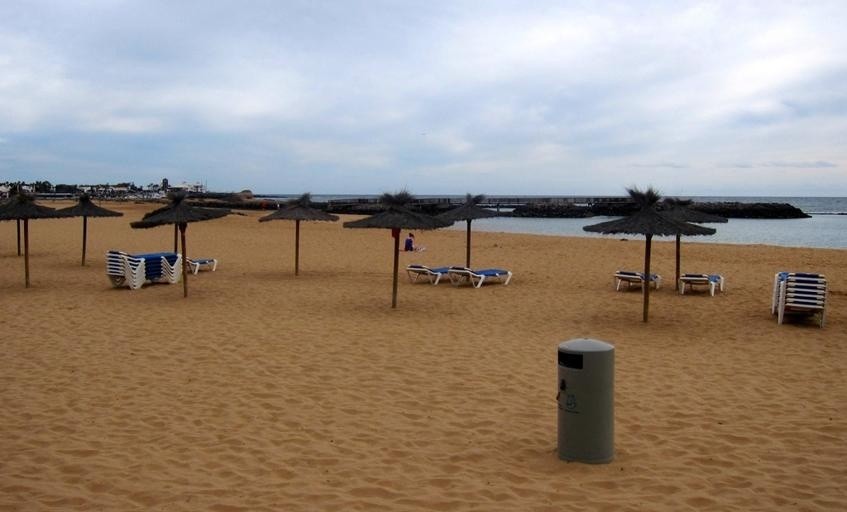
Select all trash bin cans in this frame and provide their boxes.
[558,338,615,464]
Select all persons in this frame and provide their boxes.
[405,232,421,251]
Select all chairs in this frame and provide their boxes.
[105,250,182,290]
[406,264,450,286]
[180,256,217,276]
[680,273,724,296]
[772,271,828,328]
[448,266,512,289]
[614,271,661,294]
